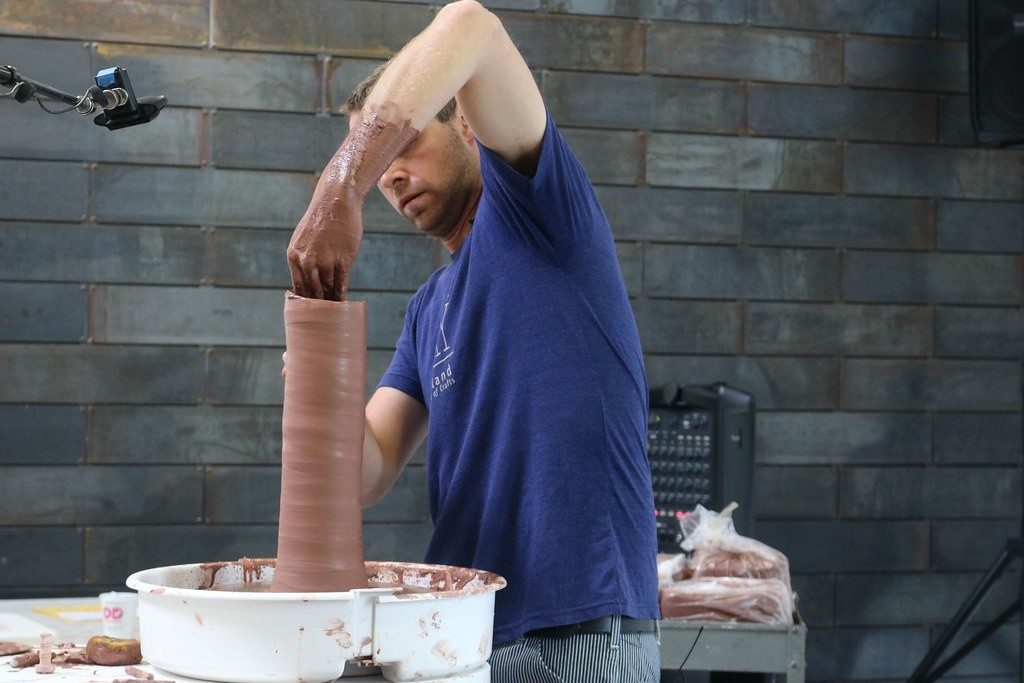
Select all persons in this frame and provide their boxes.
[282,1,664,683]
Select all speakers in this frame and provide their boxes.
[967,0,1024,150]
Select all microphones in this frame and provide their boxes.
[79,65,169,130]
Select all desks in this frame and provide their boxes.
[658,609,808,683]
[0,597,491,683]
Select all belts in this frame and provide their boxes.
[521,615,656,645]
